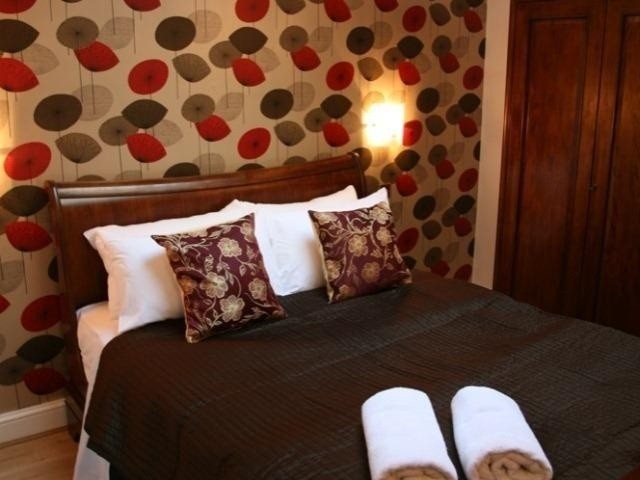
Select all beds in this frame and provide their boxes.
[45,149,640,480]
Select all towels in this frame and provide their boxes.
[361,386,459,480]
[451,386,554,480]
